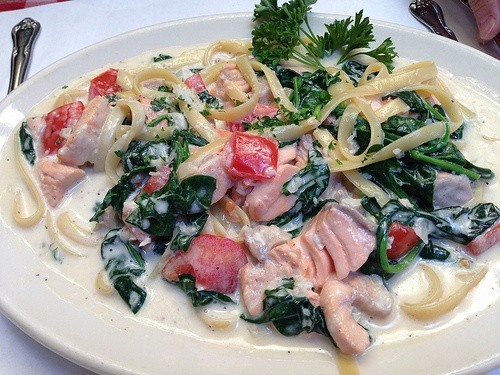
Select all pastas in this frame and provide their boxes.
[0,0,500,354]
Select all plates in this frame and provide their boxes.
[0,12,500,375]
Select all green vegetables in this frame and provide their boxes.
[19,0,500,351]
